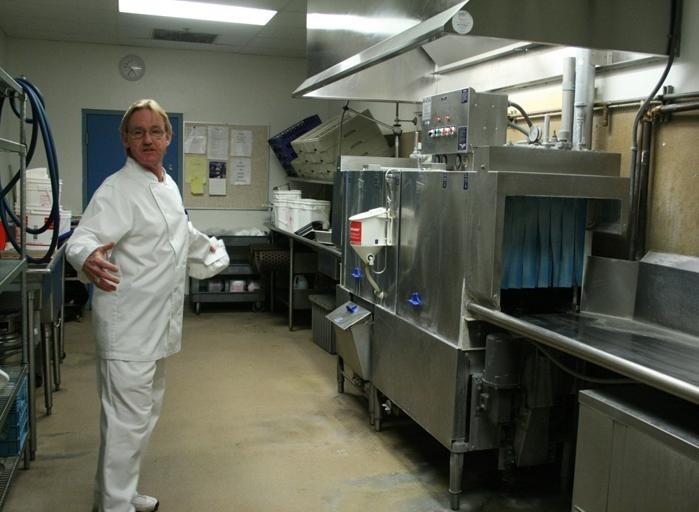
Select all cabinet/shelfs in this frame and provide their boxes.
[190,232,268,305]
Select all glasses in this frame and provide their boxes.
[126,126,169,139]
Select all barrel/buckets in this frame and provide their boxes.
[15,175,62,206]
[59,210,71,236]
[294,272,308,289]
[14,207,57,258]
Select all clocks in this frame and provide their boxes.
[119,54,146,81]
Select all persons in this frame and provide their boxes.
[65,99,219,512]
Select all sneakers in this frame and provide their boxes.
[131,493,159,512]
